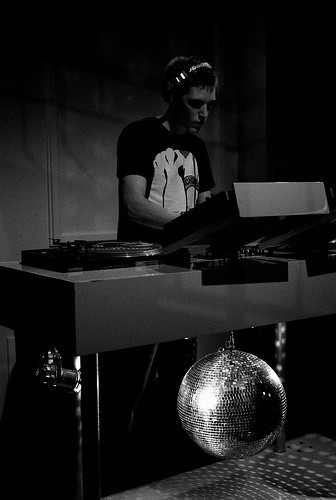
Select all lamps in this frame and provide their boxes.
[177,332,286,459]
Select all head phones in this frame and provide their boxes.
[165,62,212,92]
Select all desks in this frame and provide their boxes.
[0,257,336,355]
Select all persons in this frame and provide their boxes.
[105,54,219,441]
